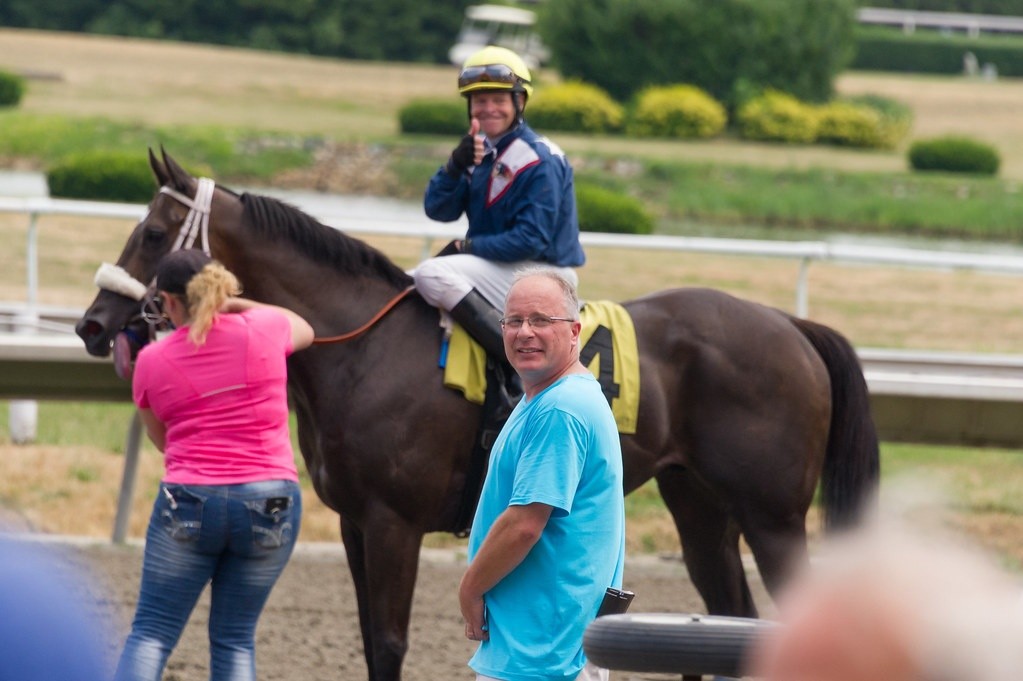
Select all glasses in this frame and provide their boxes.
[457,64,532,86]
[498,315,575,330]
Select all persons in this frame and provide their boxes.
[458,266,626,681]
[109,247,315,681]
[415,45,586,374]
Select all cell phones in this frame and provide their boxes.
[153,328,175,340]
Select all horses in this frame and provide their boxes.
[74,144,881,681]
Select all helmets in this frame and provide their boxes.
[458,45,533,101]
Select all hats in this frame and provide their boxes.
[156,248,210,292]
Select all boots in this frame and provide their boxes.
[448,287,524,402]
